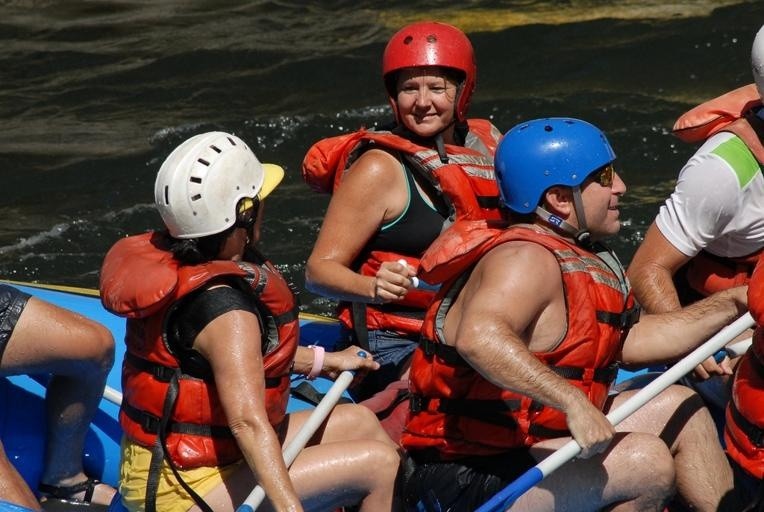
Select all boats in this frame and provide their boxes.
[0,277,652,512]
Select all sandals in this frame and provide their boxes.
[37,472,111,512]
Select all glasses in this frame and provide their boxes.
[586,162,614,188]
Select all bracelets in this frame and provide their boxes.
[308,345,323,380]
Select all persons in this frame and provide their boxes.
[626,25,764,449]
[401,118,747,512]
[0,285,117,512]
[723,249,764,481]
[100,130,401,512]
[305,21,504,395]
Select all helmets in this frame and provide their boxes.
[382,21,478,127]
[493,117,617,215]
[154,130,286,240]
